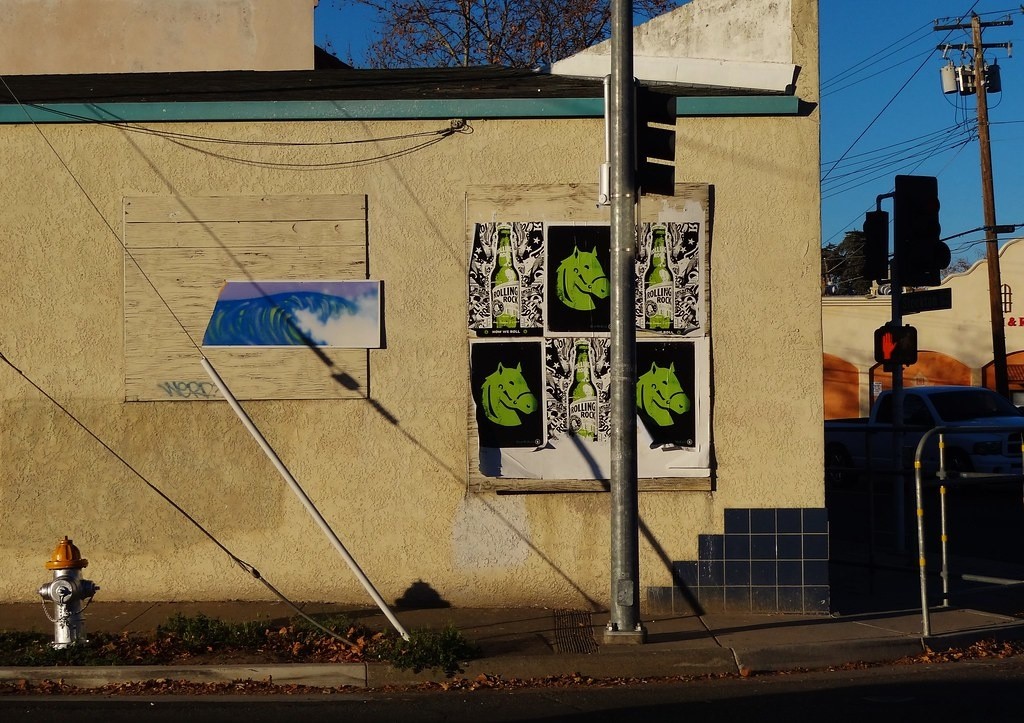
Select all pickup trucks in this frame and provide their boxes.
[823,385,1024,525]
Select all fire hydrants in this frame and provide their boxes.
[38,533,101,654]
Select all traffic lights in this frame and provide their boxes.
[633,77,677,198]
[873,322,917,367]
[894,175,950,286]
